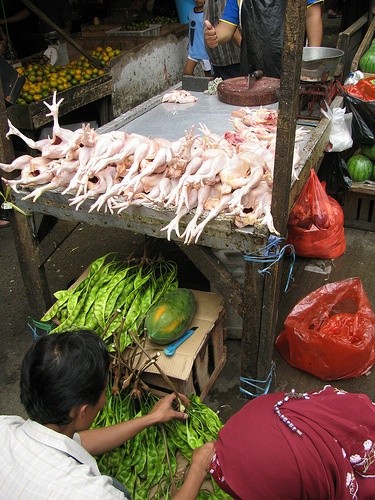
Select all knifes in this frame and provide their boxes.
[247,70,263,89]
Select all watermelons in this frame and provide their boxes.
[344,137,375,182]
[359,38,375,74]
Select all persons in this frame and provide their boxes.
[0,328,219,500]
[174,0,325,79]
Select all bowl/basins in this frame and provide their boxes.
[301,46,344,79]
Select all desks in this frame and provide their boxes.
[0,82,344,401]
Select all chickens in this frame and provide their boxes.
[162,89,198,104]
[0,91,312,246]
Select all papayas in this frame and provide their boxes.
[147,288,196,345]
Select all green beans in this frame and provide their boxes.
[82,388,238,500]
[39,251,178,353]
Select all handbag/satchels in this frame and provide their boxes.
[322,153,352,192]
[285,169,345,259]
[335,75,375,145]
[275,277,375,381]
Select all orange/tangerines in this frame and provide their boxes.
[14,46,119,105]
[120,15,180,31]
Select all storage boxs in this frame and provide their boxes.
[52,267,226,404]
[209,249,245,338]
[82,20,162,36]
[343,180,375,232]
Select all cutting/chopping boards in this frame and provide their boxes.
[217,76,280,106]
